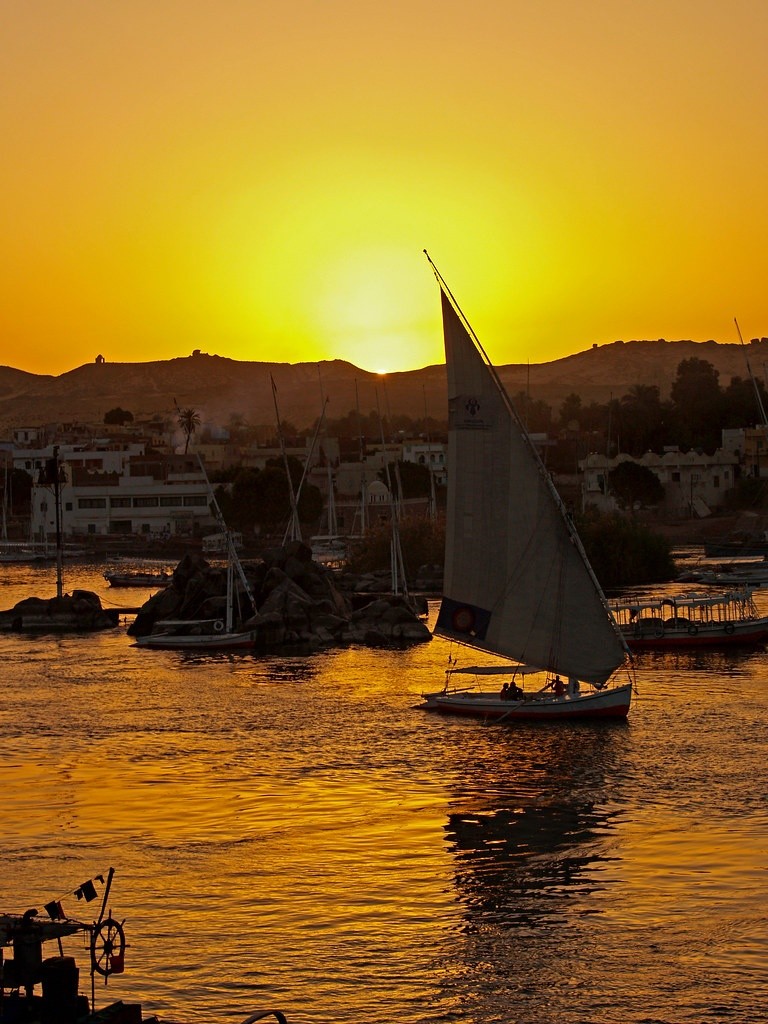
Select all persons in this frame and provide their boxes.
[499,681,523,702]
[550,676,564,697]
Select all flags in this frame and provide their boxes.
[81,880,98,902]
[74,889,83,900]
[43,900,60,920]
[55,901,66,921]
[95,875,105,884]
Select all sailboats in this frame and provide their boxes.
[352,386,429,617]
[1,440,121,632]
[308,441,378,553]
[267,371,352,575]
[127,524,256,650]
[407,246,634,720]
[101,571,173,587]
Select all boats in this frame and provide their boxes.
[602,588,768,650]
[1,866,286,1024]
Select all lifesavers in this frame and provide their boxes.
[724,623,735,635]
[688,625,699,636]
[654,630,664,638]
[213,621,224,631]
[634,631,643,640]
